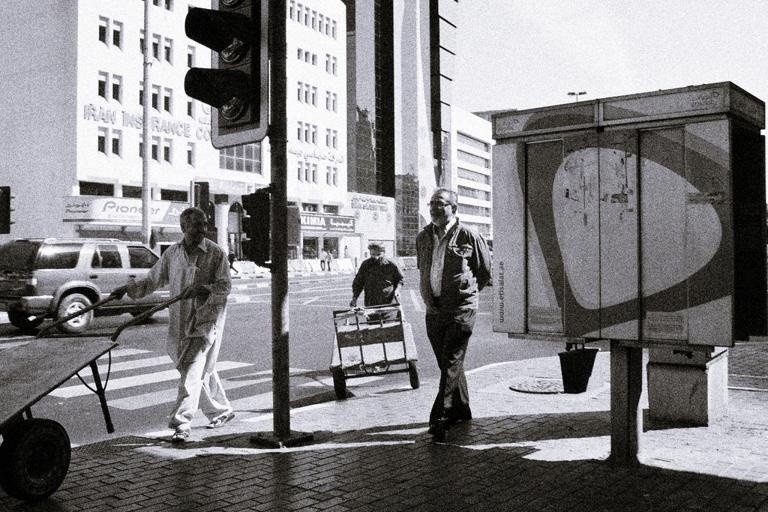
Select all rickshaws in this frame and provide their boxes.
[331,292,422,402]
[0,289,181,497]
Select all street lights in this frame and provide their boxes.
[567,90,588,101]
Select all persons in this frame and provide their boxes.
[347,242,405,374]
[108,206,235,441]
[317,244,351,272]
[415,189,492,436]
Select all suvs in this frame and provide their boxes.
[0,233,171,333]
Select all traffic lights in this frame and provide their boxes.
[0,184,16,235]
[240,194,270,261]
[183,0,267,150]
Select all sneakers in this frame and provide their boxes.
[172,431,190,441]
[206,413,235,428]
